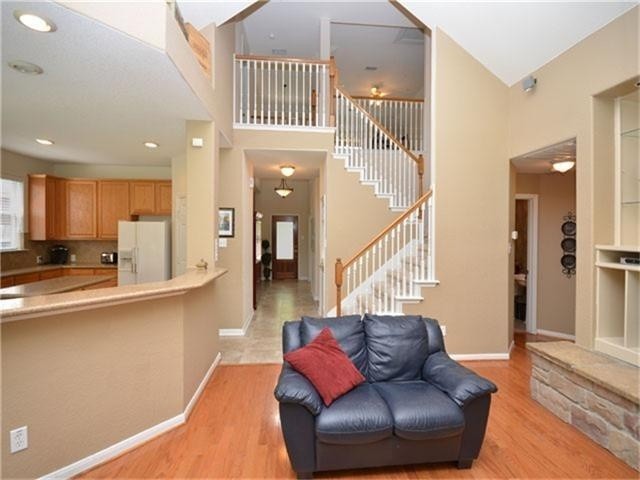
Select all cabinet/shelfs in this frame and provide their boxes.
[133,179,173,218]
[29,174,64,242]
[66,179,131,242]
[591,90,640,368]
[2,268,119,285]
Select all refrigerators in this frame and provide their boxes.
[117,216,170,287]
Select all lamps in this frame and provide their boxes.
[275,178,293,198]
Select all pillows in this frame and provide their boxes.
[283,326,372,406]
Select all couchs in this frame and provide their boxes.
[275,315,499,480]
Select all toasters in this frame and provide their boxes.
[100,252,117,264]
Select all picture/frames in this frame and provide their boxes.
[217,206,235,237]
